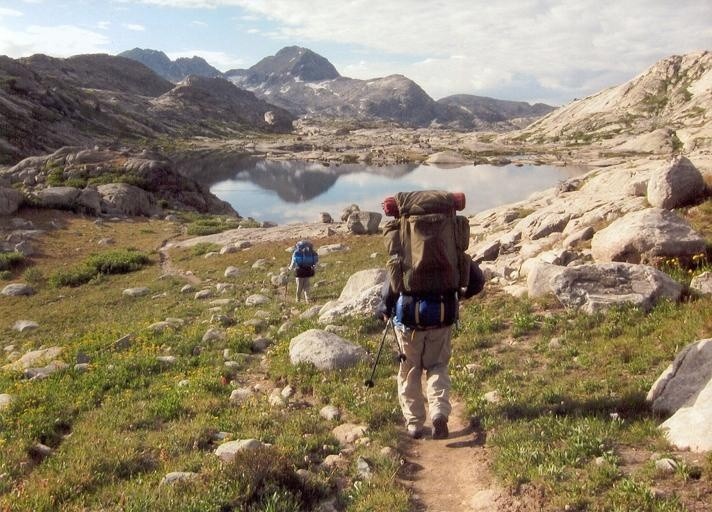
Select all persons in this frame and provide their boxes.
[376,190,484,440]
[288,241,318,306]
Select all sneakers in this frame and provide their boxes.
[409,429,422,439]
[432,413,448,439]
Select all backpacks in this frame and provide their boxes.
[382,191,472,330]
[294,243,319,277]
